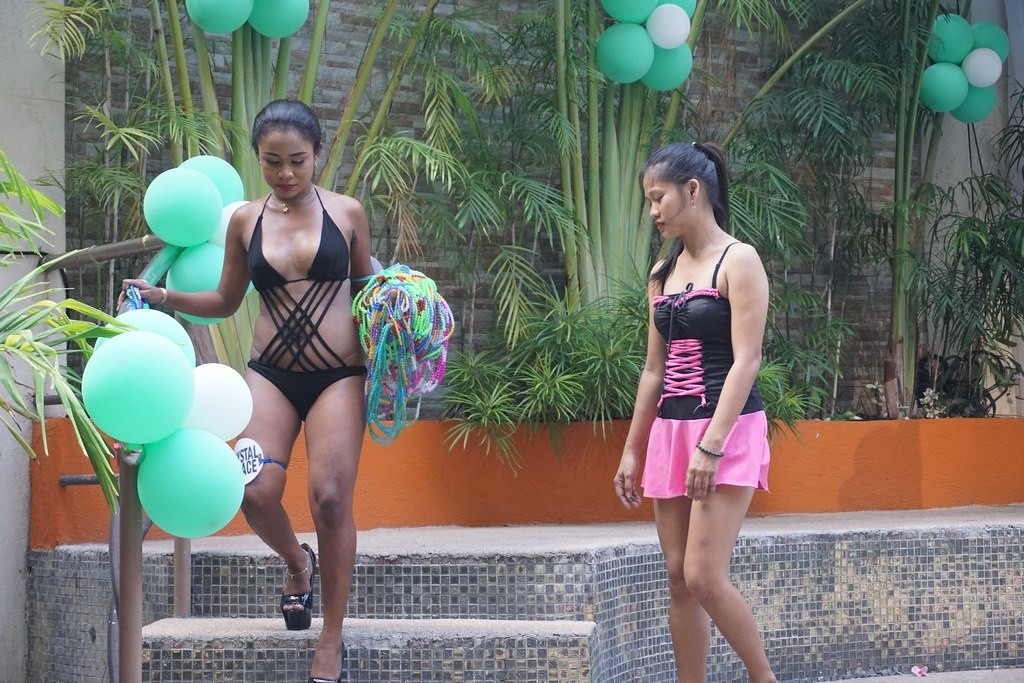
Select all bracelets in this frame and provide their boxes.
[696,441,724,456]
[156,287,167,305]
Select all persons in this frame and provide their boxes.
[614,142,778,683]
[117,98,425,683]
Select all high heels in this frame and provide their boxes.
[280,543,316,630]
[308,640,345,683]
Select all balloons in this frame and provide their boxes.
[927,13,972,65]
[950,82,997,123]
[646,4,690,48]
[657,0,696,18]
[185,0,253,33]
[962,48,1002,88]
[247,0,309,37]
[972,21,1010,61]
[641,39,692,91]
[600,0,658,24]
[597,22,654,83]
[81,155,253,540]
[919,62,968,111]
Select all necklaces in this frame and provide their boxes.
[271,188,315,212]
